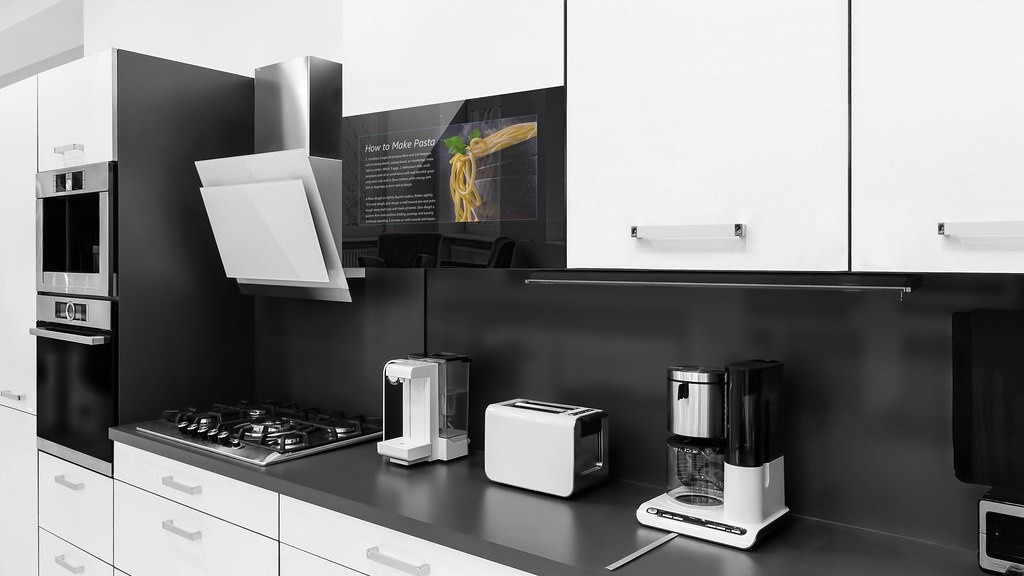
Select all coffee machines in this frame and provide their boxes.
[377,352,473,467]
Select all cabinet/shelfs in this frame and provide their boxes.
[36,47,119,174]
[112,441,280,576]
[563,0,849,276]
[37,450,114,576]
[1,405,39,575]
[847,0,1022,278]
[1,74,36,418]
[278,494,541,576]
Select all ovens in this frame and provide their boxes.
[33,158,121,300]
[29,296,121,478]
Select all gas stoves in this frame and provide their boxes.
[138,397,384,465]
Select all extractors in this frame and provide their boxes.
[193,55,362,308]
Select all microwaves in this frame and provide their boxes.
[975,485,1024,576]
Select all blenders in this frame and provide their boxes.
[635,355,796,551]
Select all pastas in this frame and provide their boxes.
[448,122,537,222]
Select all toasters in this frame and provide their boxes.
[484,398,613,498]
